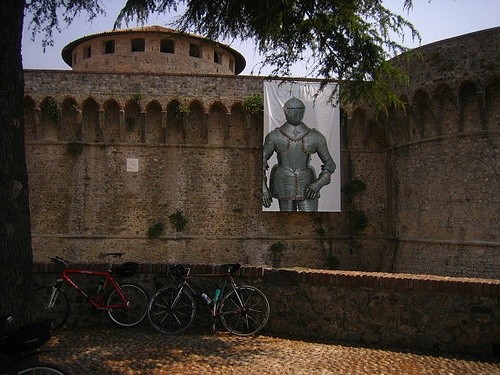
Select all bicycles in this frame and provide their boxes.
[1,312,70,374]
[25,250,150,328]
[146,261,272,338]
